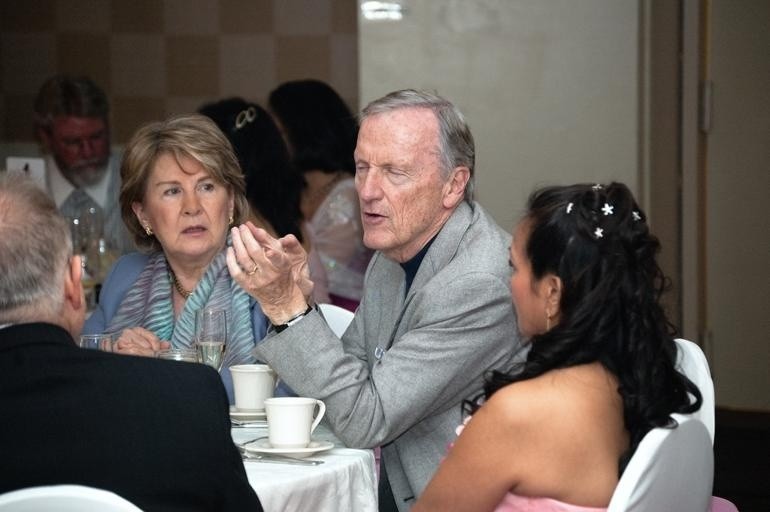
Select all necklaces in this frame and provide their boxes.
[169,272,194,301]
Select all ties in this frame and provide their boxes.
[69,189,93,237]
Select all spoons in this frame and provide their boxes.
[231,420,266,426]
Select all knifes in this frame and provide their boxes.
[240,455,324,466]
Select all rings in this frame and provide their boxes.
[247,265,260,278]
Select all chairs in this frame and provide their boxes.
[607,414,714,512]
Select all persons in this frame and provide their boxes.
[226,87,534,512]
[266,78,377,311]
[26,75,143,252]
[75,112,300,407]
[197,97,332,307]
[408,182,737,512]
[0,171,265,512]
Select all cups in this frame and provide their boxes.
[195,309,225,374]
[264,397,326,448]
[230,364,279,411]
[77,334,112,351]
[160,349,201,364]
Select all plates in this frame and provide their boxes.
[238,437,335,458]
[230,404,267,420]
